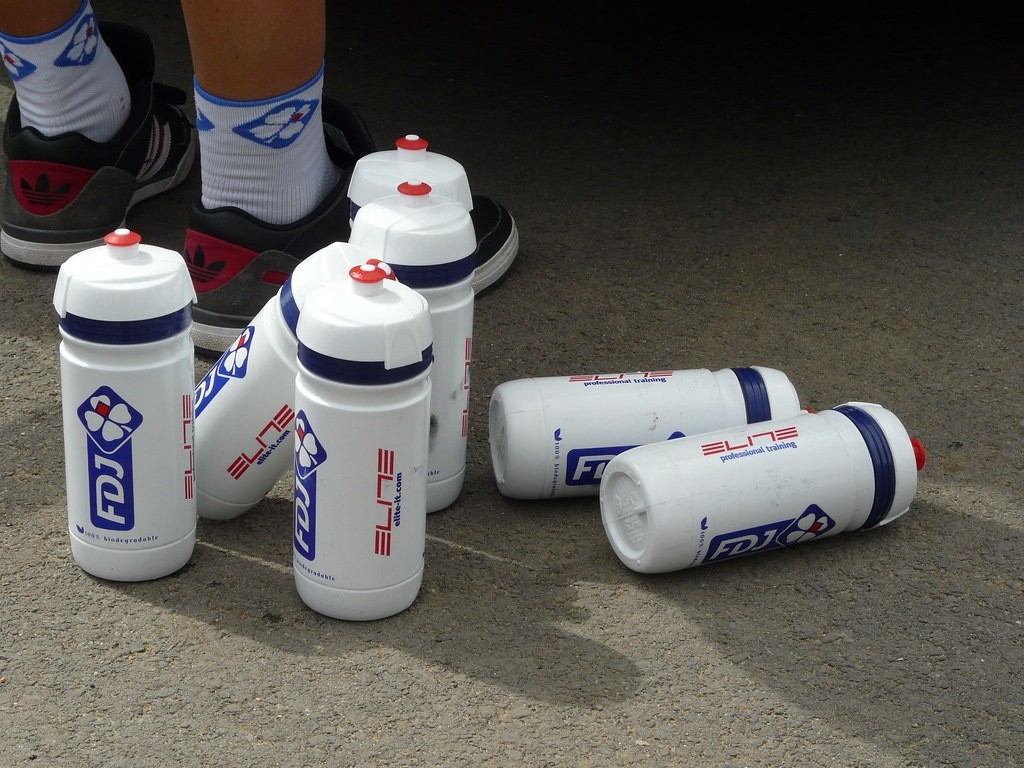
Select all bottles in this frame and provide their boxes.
[51,229,198,581]
[489,365,814,501]
[292,263,425,622]
[351,181,477,514]
[194,242,399,522]
[599,401,927,575]
[345,133,477,227]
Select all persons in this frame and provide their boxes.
[1,0,521,354]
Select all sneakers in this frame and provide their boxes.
[0,20,195,272]
[181,96,518,360]
